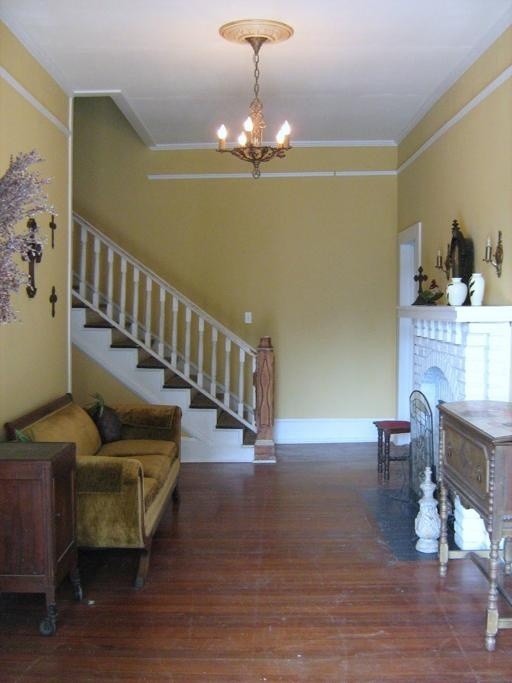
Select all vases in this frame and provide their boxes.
[446,271,485,307]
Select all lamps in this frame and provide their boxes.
[482,230,504,278]
[213,18,296,178]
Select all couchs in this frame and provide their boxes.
[4,390,182,588]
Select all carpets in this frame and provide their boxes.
[361,485,461,562]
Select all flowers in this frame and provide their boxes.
[1,148,59,325]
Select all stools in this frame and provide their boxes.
[371,421,411,481]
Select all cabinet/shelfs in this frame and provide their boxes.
[0,440,84,639]
[435,400,512,653]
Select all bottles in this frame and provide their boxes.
[468,273,485,306]
[447,276,467,306]
